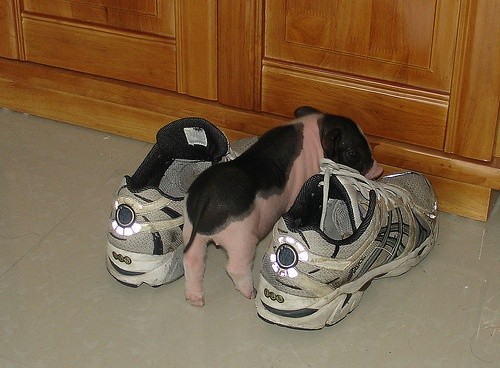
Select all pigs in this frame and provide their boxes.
[182,105,384,306]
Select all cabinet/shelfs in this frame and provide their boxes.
[1,0,500,221]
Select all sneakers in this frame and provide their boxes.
[256,159,439,331]
[106,117,262,287]
